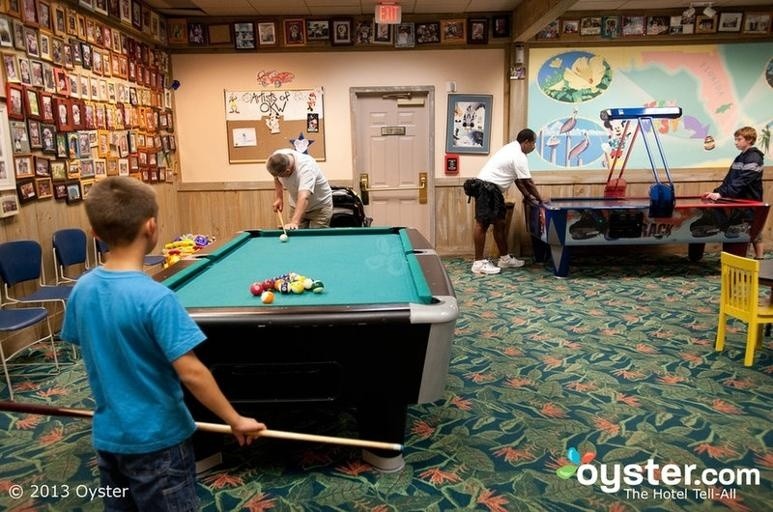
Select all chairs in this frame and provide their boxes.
[716,251,772,368]
[0,231,168,400]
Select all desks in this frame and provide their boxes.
[522,193,773,279]
[153,225,462,477]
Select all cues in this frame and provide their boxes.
[276,208,287,235]
[0,395,403,454]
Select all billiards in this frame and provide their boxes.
[278,232,290,241]
[250,280,263,294]
[261,292,274,303]
[264,271,326,294]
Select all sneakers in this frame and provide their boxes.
[497,254,526,268]
[471,259,503,275]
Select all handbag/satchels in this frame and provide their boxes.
[463,179,483,197]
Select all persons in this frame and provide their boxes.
[167,16,483,49]
[56,175,266,511]
[266,147,334,232]
[468,126,552,275]
[701,125,766,261]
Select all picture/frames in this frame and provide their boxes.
[446,91,495,155]
[534,8,773,42]
